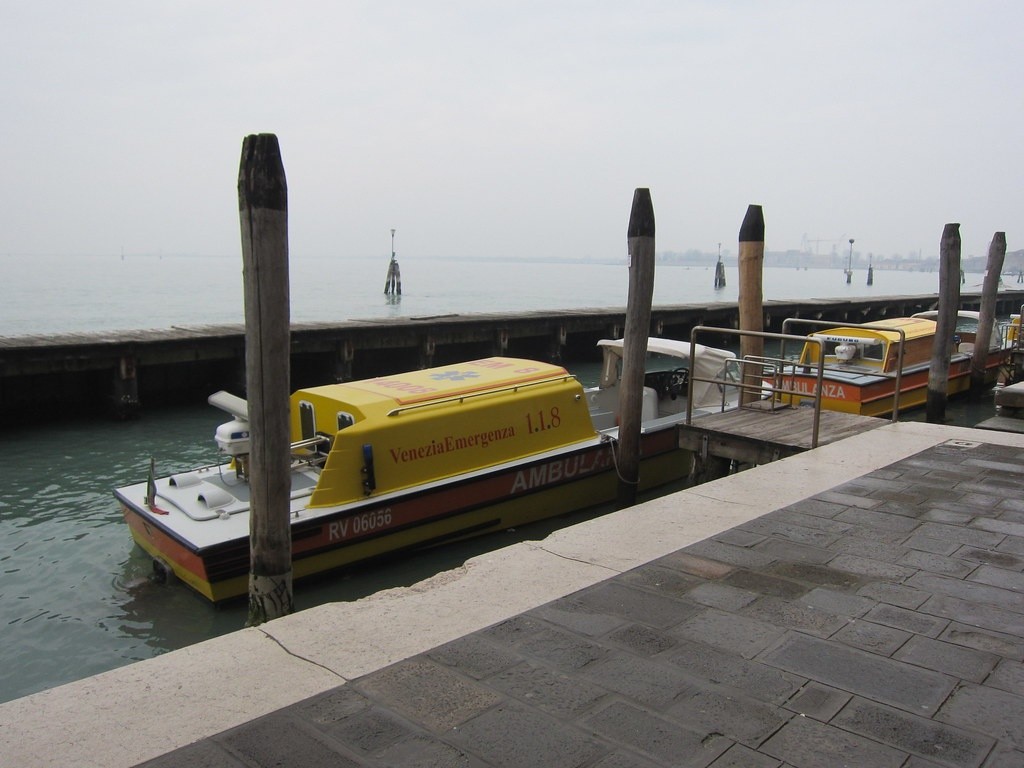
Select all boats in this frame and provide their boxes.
[109,335,773,601]
[761,304,1024,417]
